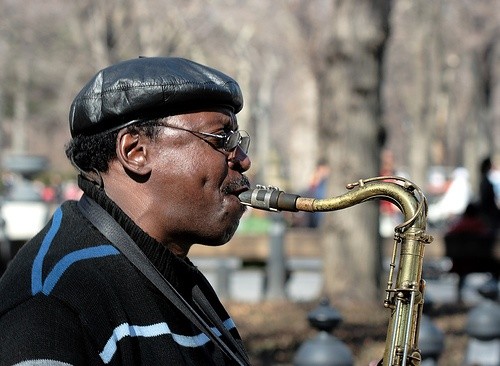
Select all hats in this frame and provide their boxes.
[69,56,244,143]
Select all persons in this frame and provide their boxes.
[0,56,379,366]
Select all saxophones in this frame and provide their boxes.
[235,175,433,366]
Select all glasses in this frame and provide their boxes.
[131,122,251,157]
